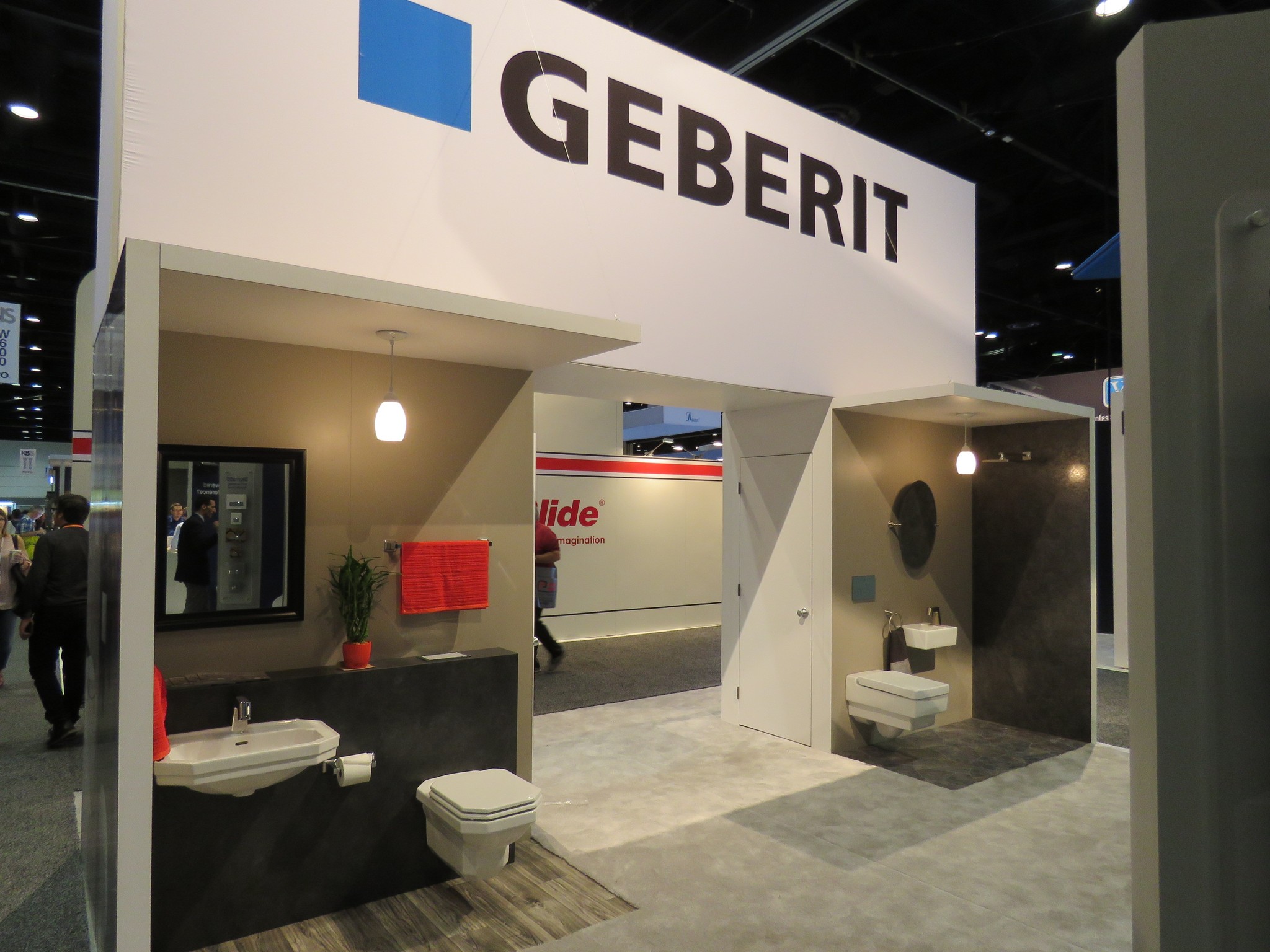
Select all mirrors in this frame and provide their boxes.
[157,443,306,628]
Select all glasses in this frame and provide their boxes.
[50,508,57,513]
[37,513,42,517]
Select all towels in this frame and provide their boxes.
[886,627,909,671]
[399,540,489,615]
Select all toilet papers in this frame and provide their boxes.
[336,752,373,787]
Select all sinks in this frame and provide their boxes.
[154,718,340,798]
[895,622,957,649]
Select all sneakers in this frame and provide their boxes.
[0,670,5,687]
[46,724,84,750]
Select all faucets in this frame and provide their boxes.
[927,606,942,626]
[231,694,251,733]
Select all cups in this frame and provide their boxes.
[8,549,23,564]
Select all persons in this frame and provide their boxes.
[535,504,566,673]
[19,494,90,748]
[0,508,32,686]
[166,502,185,536]
[174,497,216,612]
[5,509,22,535]
[167,507,187,551]
[15,505,47,536]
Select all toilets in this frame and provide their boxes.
[845,669,950,739]
[416,768,543,876]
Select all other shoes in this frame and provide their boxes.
[534,653,566,673]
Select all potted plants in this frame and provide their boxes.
[328,544,397,672]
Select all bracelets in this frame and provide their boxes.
[19,559,24,566]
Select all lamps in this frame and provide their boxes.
[374,329,410,443]
[957,412,977,474]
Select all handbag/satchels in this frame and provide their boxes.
[11,563,27,619]
